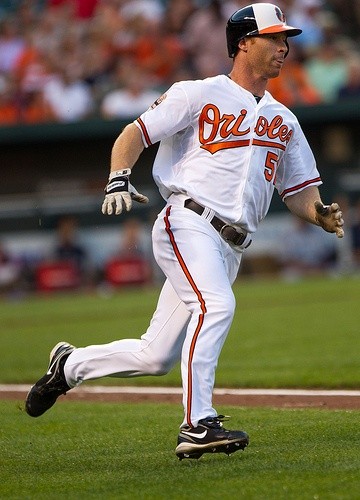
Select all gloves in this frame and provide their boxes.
[314,201,345,239]
[101,169,148,214]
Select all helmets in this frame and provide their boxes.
[225,3,302,58]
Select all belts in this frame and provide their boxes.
[184,199,252,250]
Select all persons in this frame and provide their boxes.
[25,3,345,458]
[0,0,360,290]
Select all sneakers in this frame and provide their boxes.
[176,415,247,458]
[25,341,77,417]
